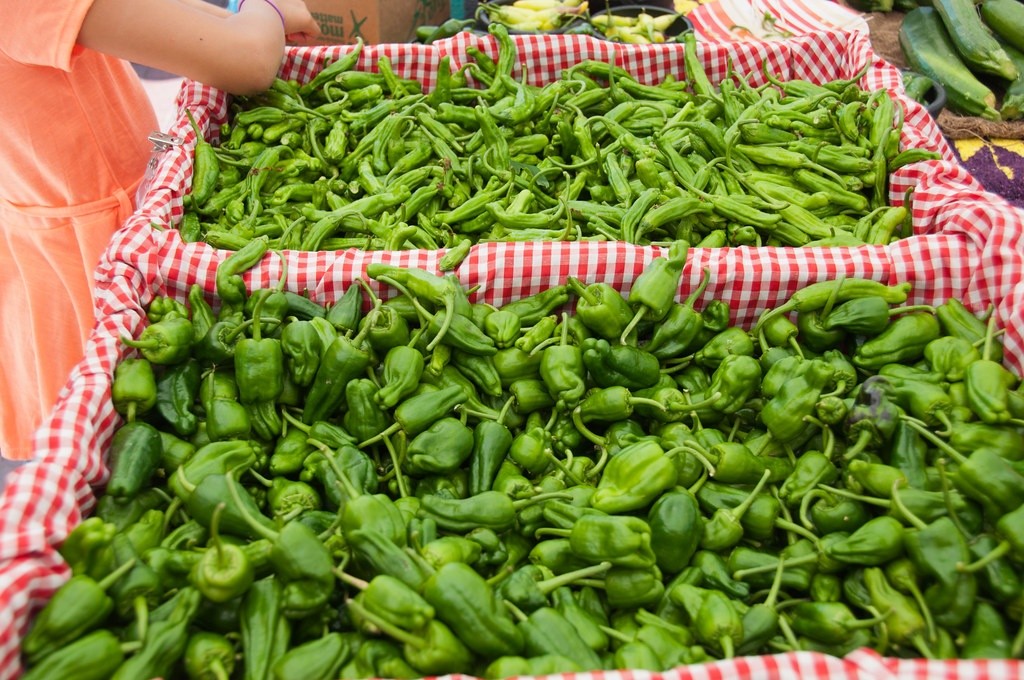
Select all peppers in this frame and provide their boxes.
[20,0,1024,680]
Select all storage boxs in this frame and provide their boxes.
[285,0,450,51]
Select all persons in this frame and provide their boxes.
[0,0,323,467]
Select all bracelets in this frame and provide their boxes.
[238,0,284,27]
